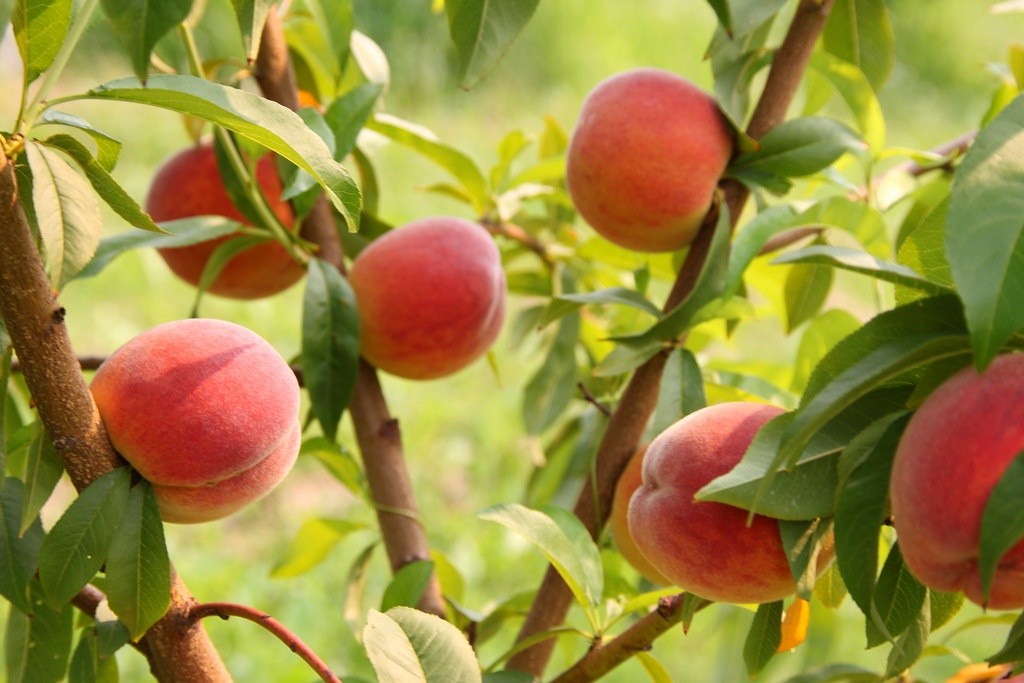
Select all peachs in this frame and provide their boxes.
[345,213,508,379]
[608,401,839,604]
[886,350,1024,683]
[145,133,313,299]
[567,69,736,253]
[89,316,302,524]
